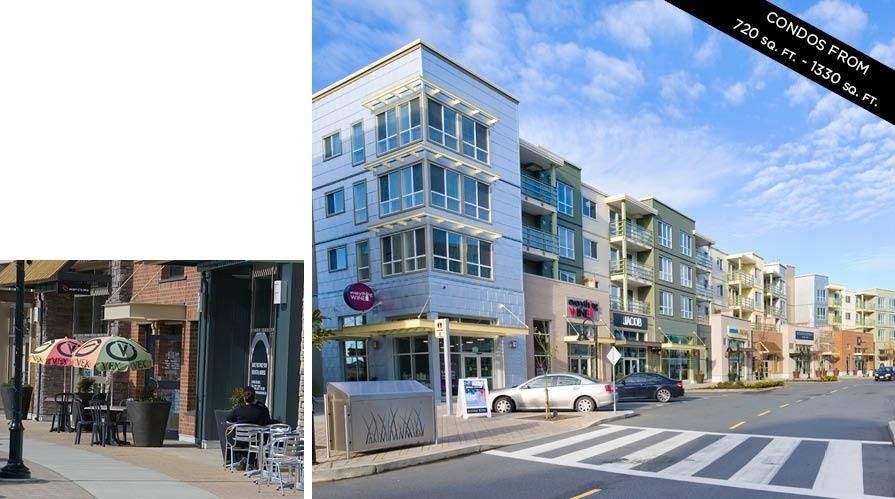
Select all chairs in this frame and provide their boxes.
[50,393,74,433]
[224,419,304,496]
[120,398,139,444]
[73,396,101,445]
[91,392,119,443]
[89,399,120,447]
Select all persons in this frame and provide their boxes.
[225,385,268,470]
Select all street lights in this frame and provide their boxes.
[798,345,813,380]
[577,318,599,381]
[726,339,741,382]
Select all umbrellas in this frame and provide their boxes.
[27,336,83,396]
[71,333,153,408]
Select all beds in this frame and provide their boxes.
[44,395,82,432]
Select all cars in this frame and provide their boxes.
[614,372,686,403]
[487,372,621,415]
[875,366,895,381]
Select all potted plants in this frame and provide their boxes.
[214,385,245,462]
[0,377,33,420]
[71,377,106,432]
[125,380,172,446]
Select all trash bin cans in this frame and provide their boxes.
[324,380,437,459]
